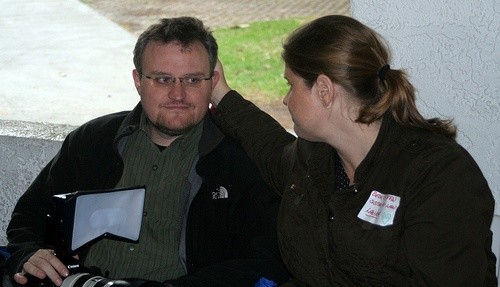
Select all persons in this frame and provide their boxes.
[0,15,297,287]
[211,15,498,286]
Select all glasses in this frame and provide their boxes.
[141,73,211,87]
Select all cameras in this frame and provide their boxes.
[20,183,146,287]
